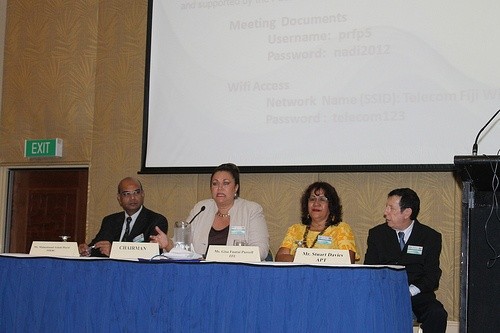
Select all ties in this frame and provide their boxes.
[399,232,406,251]
[120,217,132,241]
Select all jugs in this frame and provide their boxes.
[173,221,191,251]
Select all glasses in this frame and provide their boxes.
[309,196,328,203]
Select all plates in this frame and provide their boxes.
[164,253,201,261]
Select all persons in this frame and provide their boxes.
[275,182,360,264]
[150,163,271,261]
[79,176,169,257]
[362,188,448,333]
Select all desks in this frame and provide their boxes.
[0,254,413,333]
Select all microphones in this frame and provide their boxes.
[189,206,205,224]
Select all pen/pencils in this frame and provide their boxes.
[89,246,95,250]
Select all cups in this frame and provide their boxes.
[294,240,307,256]
[59,235,71,242]
[233,240,247,246]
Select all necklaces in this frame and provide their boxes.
[217,212,230,218]
[303,225,329,248]
